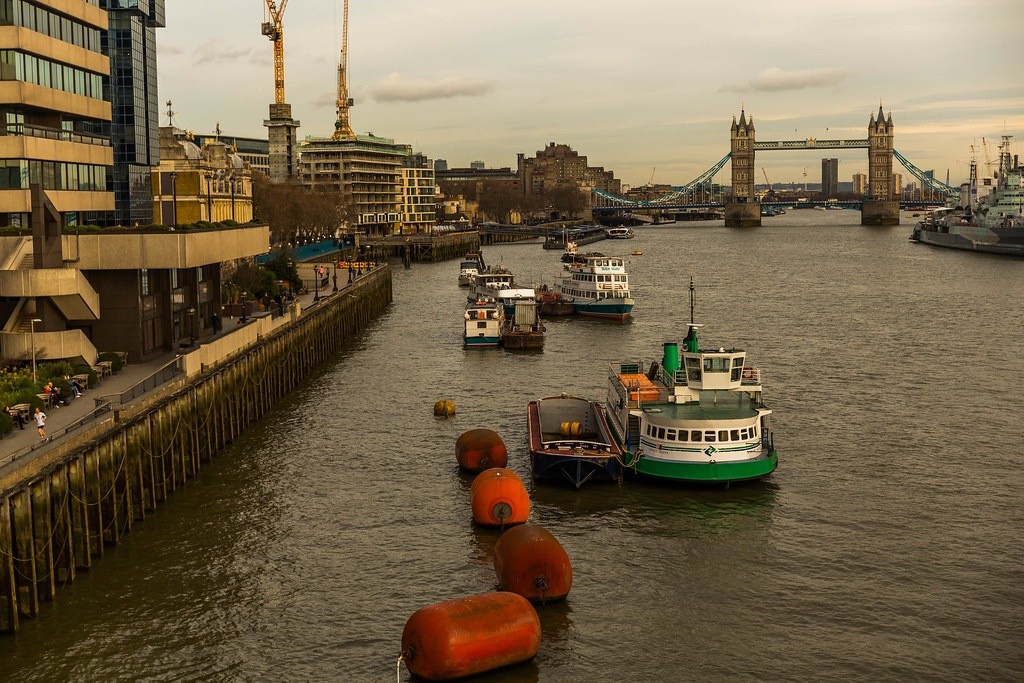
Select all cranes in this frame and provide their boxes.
[970,137,999,177]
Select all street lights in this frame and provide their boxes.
[347,239,386,283]
[240,290,247,323]
[31,319,42,383]
[313,265,320,301]
[333,258,338,291]
[187,306,196,348]
[278,280,283,296]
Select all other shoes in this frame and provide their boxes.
[54,393,83,409]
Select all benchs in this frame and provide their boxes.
[90,366,102,382]
[97,361,113,380]
[72,374,89,391]
[9,403,31,425]
[99,352,128,366]
[36,394,50,410]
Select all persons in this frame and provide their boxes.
[3,406,28,430]
[262,293,269,313]
[63,374,85,398]
[318,266,331,278]
[33,407,49,443]
[44,382,71,408]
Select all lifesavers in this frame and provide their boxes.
[743,367,753,378]
[475,302,486,305]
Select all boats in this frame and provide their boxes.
[604,272,781,484]
[631,251,642,255]
[608,225,634,239]
[535,287,574,318]
[462,294,507,348]
[562,242,604,264]
[458,259,482,285]
[503,301,546,348]
[911,154,1024,257]
[526,392,622,488]
[467,266,544,318]
[554,243,635,319]
